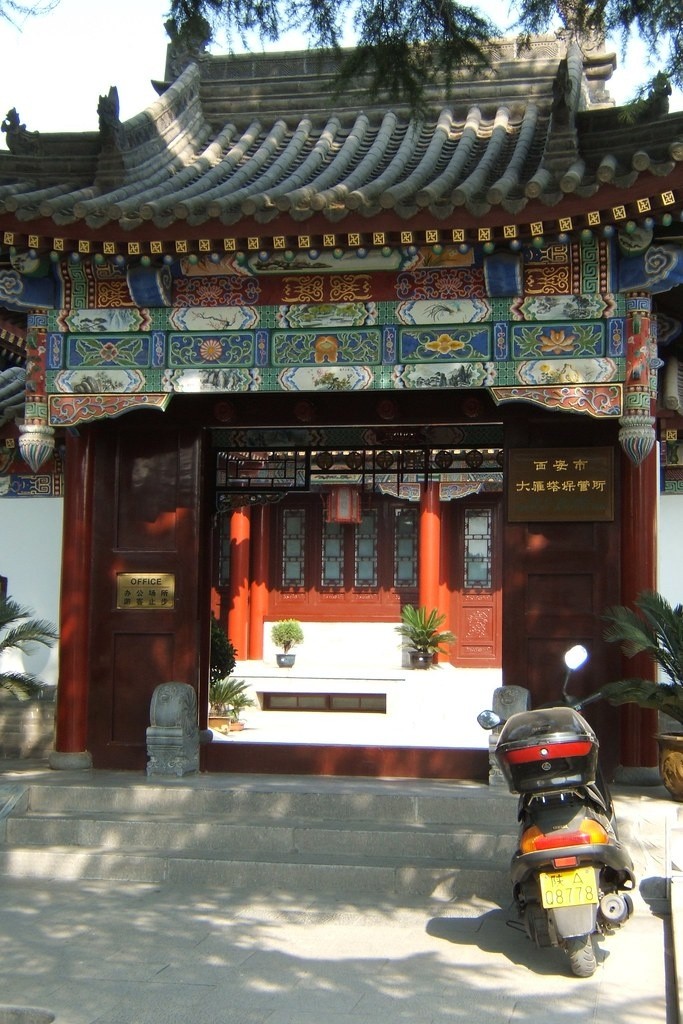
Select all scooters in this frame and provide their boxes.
[478,642,636,979]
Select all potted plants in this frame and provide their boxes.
[271,617,303,668]
[597,588,683,802]
[209,680,248,733]
[227,697,245,731]
[394,604,457,670]
